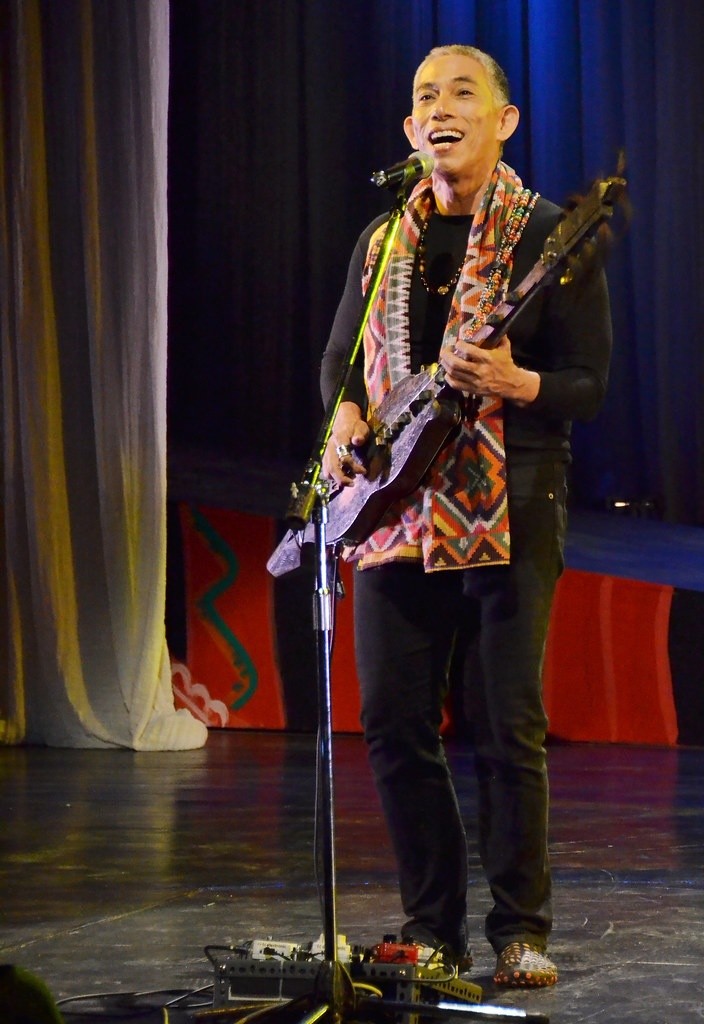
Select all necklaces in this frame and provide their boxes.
[415,206,469,297]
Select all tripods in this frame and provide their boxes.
[188,184,548,1024]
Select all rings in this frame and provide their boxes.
[335,444,353,461]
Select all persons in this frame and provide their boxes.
[319,45,615,990]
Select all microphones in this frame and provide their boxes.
[370,151,434,188]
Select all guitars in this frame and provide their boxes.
[266,175,629,579]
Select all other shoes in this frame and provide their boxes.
[395,936,473,973]
[495,942,558,986]
